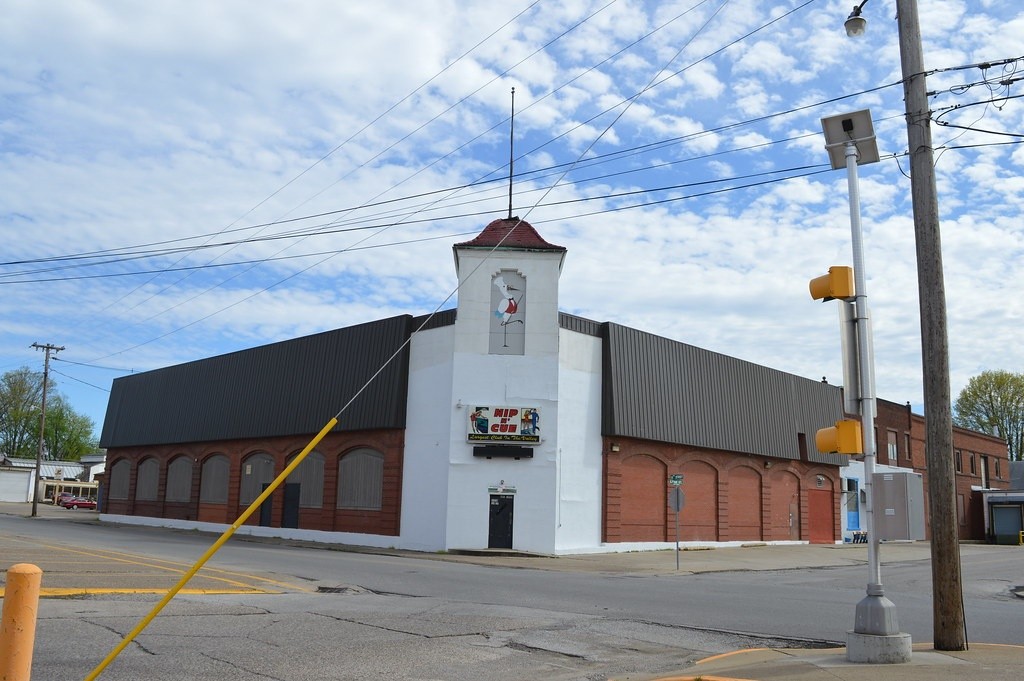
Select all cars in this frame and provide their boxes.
[51,492,76,506]
[60,496,97,510]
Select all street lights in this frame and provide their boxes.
[845,0,966,653]
[819,108,900,635]
[29,404,44,517]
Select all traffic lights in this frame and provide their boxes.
[809,266,855,304]
[816,418,863,454]
